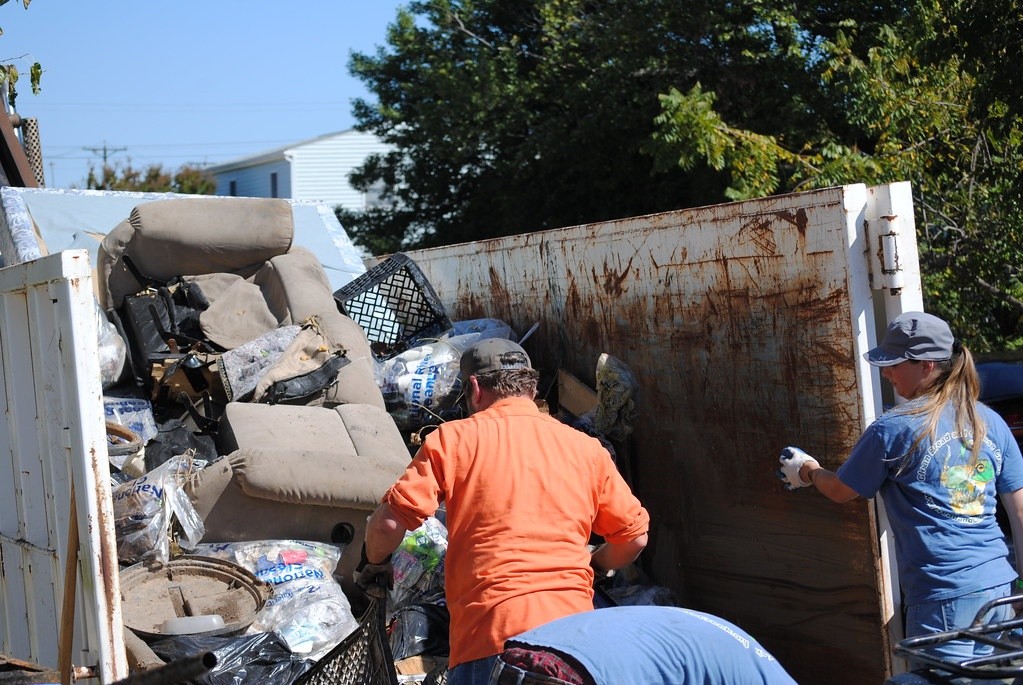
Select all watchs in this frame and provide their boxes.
[807,467,823,482]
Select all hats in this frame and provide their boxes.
[451,336,532,397]
[862,311,955,368]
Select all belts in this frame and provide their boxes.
[499,668,558,685]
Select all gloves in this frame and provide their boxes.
[589,544,616,586]
[1012,579,1023,617]
[352,541,393,601]
[776,445,819,491]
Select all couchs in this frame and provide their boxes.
[93,198,416,542]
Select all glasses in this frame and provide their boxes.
[457,390,467,412]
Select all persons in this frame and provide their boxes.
[356,337,650,685]
[772,310,1023,674]
[487,604,799,684]
